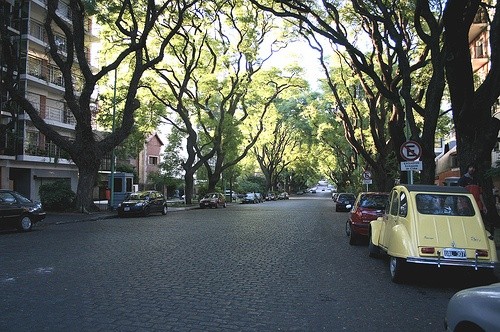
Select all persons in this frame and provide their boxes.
[458,163,488,224]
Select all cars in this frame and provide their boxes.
[0,189,45,231]
[335,193,357,213]
[118,191,168,217]
[345,191,391,245]
[368,182,498,282]
[225,186,340,205]
[200,192,227,209]
[445,279,500,332]
